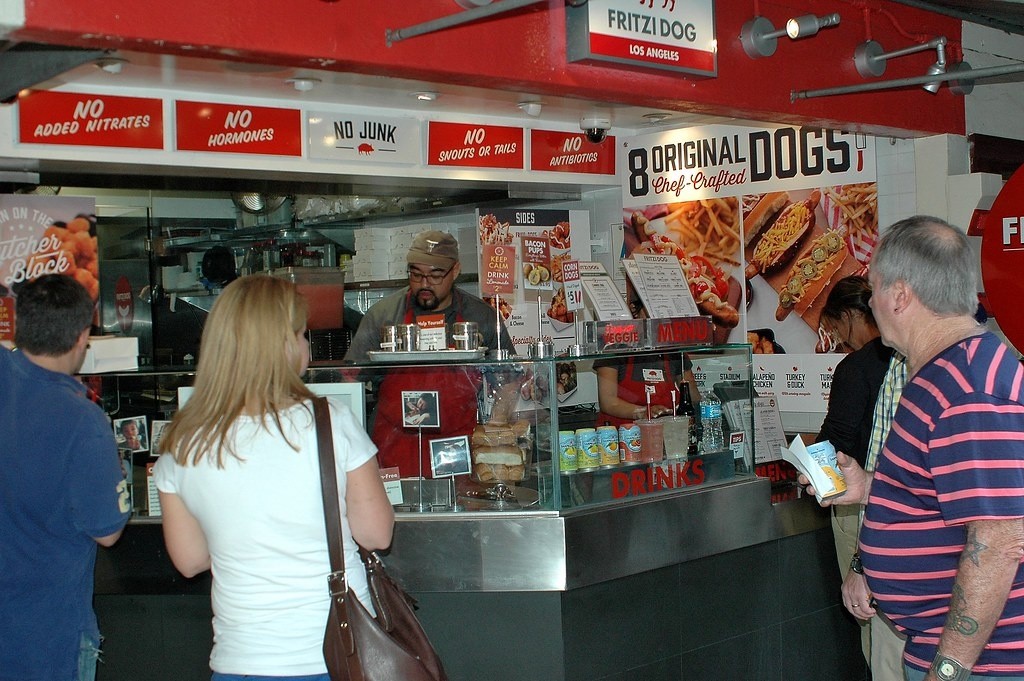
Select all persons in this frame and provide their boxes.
[404,392,436,425]
[797,215,1024,681]
[341,229,521,477]
[116,419,172,481]
[0,274,132,681]
[592,286,702,426]
[151,276,395,681]
[434,441,466,472]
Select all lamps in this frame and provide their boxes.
[740,13,840,60]
[853,35,947,94]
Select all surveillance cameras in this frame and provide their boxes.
[580,111,611,143]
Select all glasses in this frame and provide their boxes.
[407,266,454,286]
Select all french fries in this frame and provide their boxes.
[663,197,740,267]
[826,182,878,248]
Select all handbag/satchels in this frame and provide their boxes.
[312,396,448,681]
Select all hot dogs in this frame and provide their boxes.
[745,188,821,278]
[631,210,739,329]
[775,225,848,321]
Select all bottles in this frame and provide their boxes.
[228,239,324,274]
[699,388,725,453]
[675,381,699,455]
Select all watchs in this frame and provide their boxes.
[850,553,864,574]
[930,651,970,681]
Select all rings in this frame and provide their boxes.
[852,604,860,607]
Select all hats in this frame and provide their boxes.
[407,232,459,269]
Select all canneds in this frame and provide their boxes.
[806,440,848,499]
[557,422,643,471]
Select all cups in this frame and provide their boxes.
[453,322,478,350]
[396,324,416,352]
[379,326,398,352]
[323,243,336,267]
[633,419,664,464]
[659,415,691,458]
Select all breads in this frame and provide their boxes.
[471,420,531,481]
[742,191,789,245]
